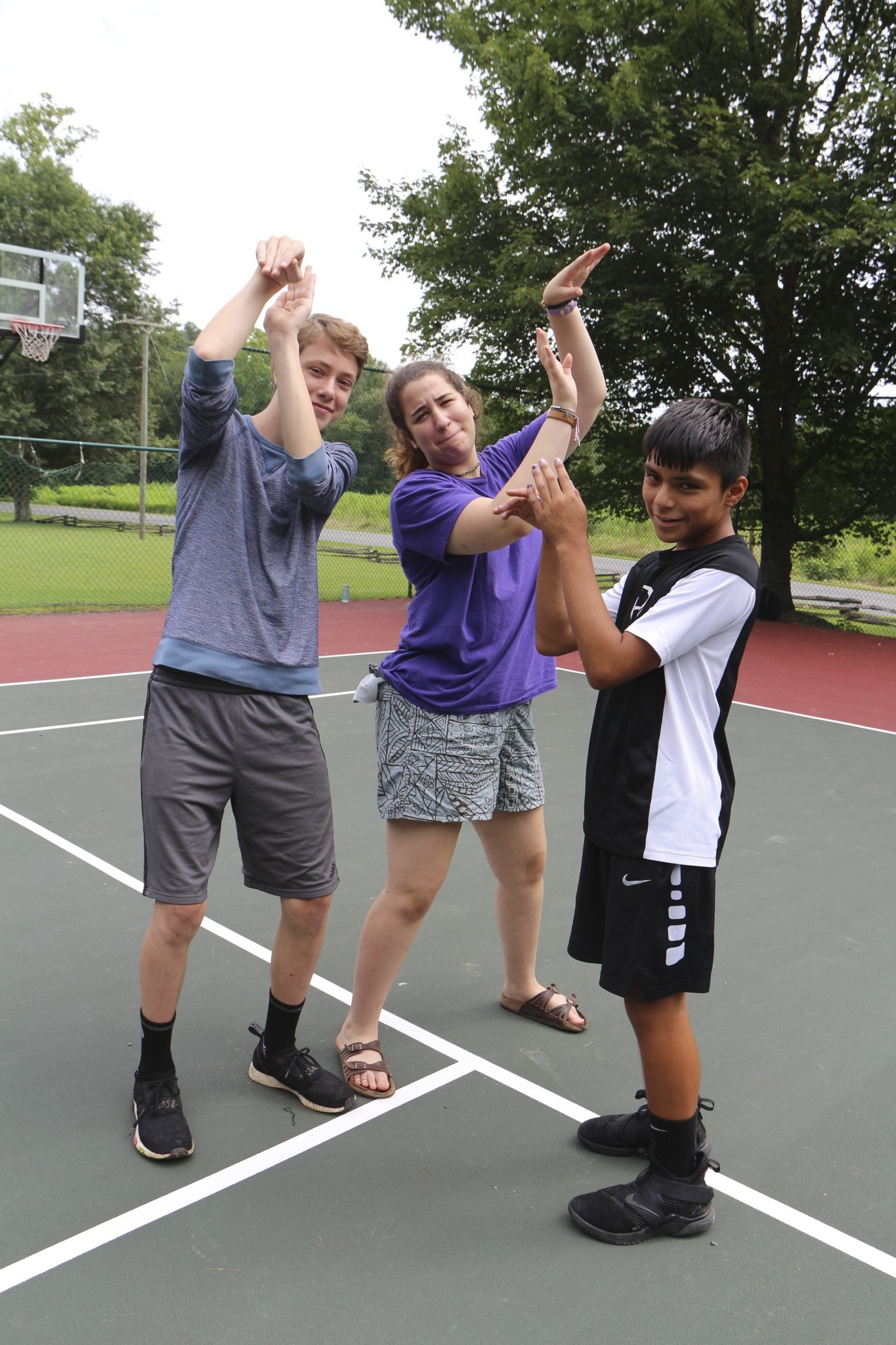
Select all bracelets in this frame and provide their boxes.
[540,298,577,316]
[547,405,581,448]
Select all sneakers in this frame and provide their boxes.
[577,1090,714,1159]
[249,1022,357,1113]
[568,1150,720,1245]
[129,1067,194,1160]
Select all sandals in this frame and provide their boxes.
[337,1041,396,1098]
[500,983,588,1033]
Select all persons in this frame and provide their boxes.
[126,235,358,1166]
[487,401,760,1246]
[332,243,614,1102]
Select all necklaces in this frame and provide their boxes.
[430,456,479,477]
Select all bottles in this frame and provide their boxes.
[342,585,349,602]
